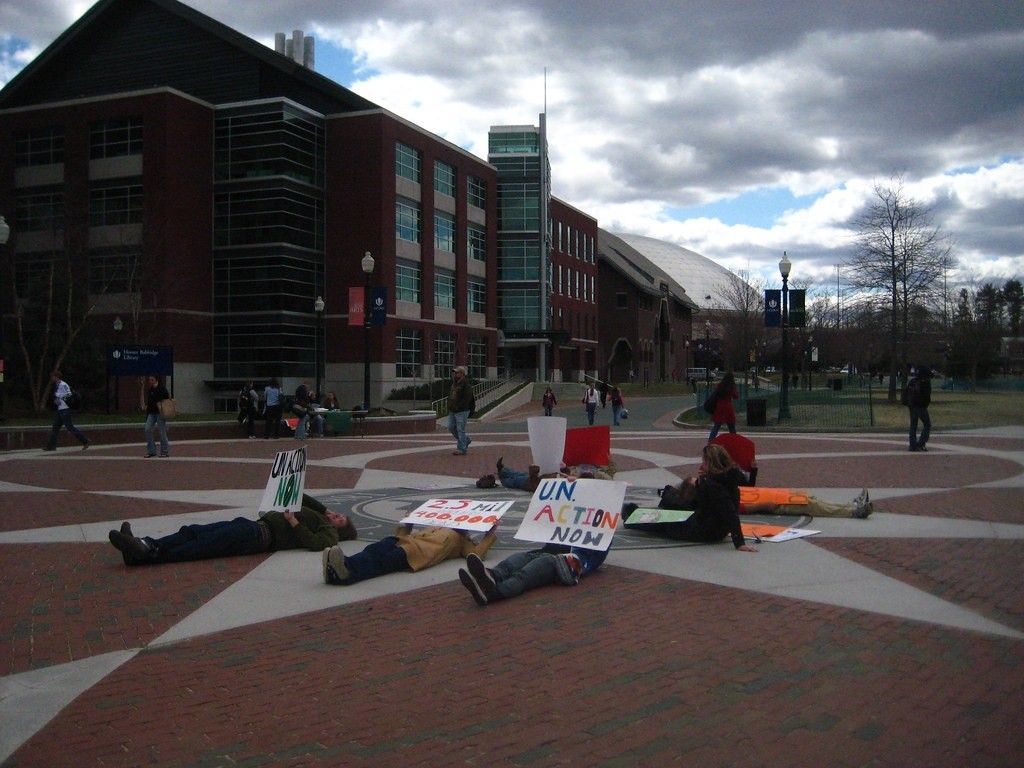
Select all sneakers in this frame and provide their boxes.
[622,502,640,528]
[459,568,489,604]
[108,521,150,566]
[466,553,496,596]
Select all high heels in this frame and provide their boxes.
[494,456,504,475]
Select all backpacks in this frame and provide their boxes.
[156,398,176,420]
[239,388,254,409]
[901,375,927,407]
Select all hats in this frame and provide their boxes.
[451,365,468,373]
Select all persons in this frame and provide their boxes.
[793,375,798,389]
[754,374,760,389]
[691,376,698,396]
[108,492,357,568]
[629,368,635,385]
[42,371,94,451]
[909,365,931,451]
[322,518,499,586]
[583,383,599,426]
[497,455,571,493]
[708,372,739,442]
[623,443,759,553]
[681,458,873,519]
[457,476,616,606]
[801,374,809,390]
[143,371,171,458]
[448,366,474,456]
[608,386,624,426]
[567,450,616,480]
[672,369,676,382]
[879,373,884,384]
[858,374,864,388]
[237,379,339,441]
[599,381,608,408]
[543,386,558,416]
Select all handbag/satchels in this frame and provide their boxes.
[704,391,717,415]
[66,391,81,408]
[582,389,589,404]
[278,386,285,401]
[292,404,308,418]
[620,409,628,419]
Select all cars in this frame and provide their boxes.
[766,367,775,372]
[840,367,857,374]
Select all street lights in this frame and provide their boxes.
[779,251,791,422]
[685,340,689,384]
[705,320,711,398]
[315,296,324,408]
[808,335,813,390]
[113,316,122,416]
[361,252,375,415]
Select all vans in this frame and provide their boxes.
[684,368,716,381]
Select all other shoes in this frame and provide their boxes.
[452,450,466,455]
[43,448,57,451]
[144,453,157,458]
[329,545,349,580]
[249,436,257,438]
[158,454,169,457]
[322,548,336,584]
[853,488,873,519]
[81,439,90,450]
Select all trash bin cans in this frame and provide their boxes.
[746,397,768,427]
[833,377,843,391]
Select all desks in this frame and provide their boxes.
[310,410,368,438]
